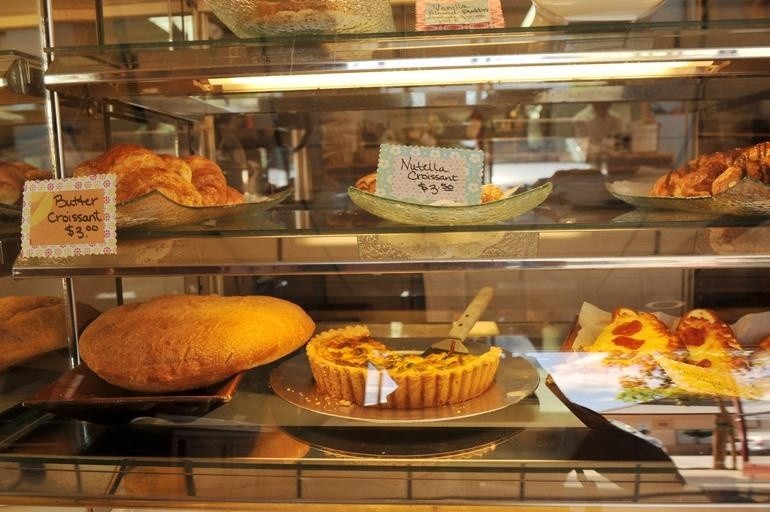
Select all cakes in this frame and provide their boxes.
[306,325,501,409]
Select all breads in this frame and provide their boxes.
[577,306,769,407]
[0,140,246,232]
[354,173,506,204]
[78,294,316,392]
[0,296,100,371]
[652,140,770,197]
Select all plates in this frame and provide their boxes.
[347,182,553,227]
[95,187,295,231]
[602,177,770,215]
[21,362,245,423]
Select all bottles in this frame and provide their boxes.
[272,111,315,205]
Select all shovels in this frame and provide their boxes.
[420,286,495,358]
